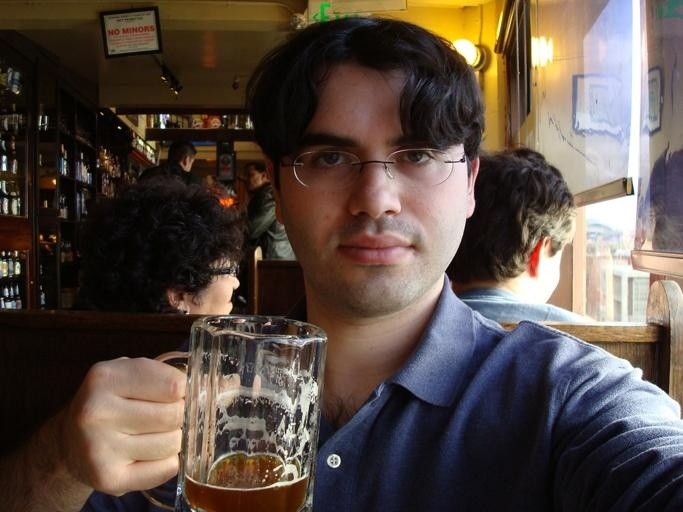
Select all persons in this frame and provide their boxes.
[71,174,240,316]
[136,139,197,184]
[238,160,299,261]
[445,146,601,325]
[1,15,683,512]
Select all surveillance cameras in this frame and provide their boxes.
[232,79,240,89]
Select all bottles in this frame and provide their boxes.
[0,65,24,311]
[60,143,85,309]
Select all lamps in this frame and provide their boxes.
[528,36,555,68]
[450,39,488,71]
[157,64,184,98]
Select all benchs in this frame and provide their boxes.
[245,245,307,319]
[1,307,682,444]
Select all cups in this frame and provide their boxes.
[134,312,325,510]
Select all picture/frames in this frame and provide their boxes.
[99,5,164,59]
[570,72,626,143]
[645,65,663,136]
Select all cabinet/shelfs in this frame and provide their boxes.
[0,27,142,316]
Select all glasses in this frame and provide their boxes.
[282,149,465,188]
